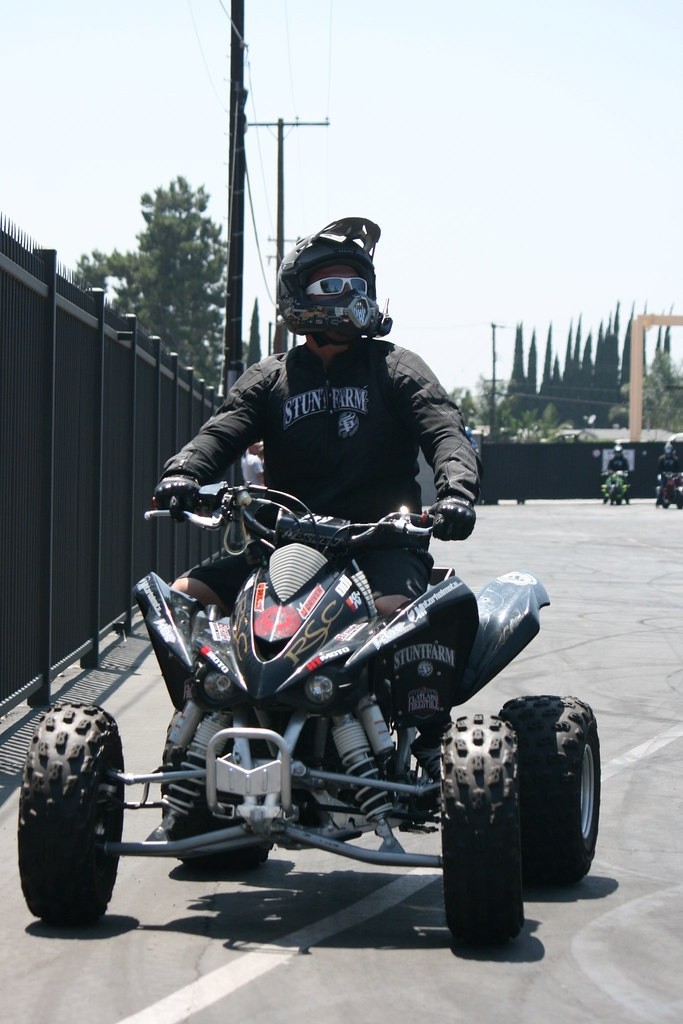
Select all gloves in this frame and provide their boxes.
[153,475,202,510]
[429,499,476,541]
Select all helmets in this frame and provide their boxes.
[277,217,393,338]
[614,444,623,457]
[664,441,673,454]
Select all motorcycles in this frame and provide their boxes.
[17,480,602,941]
[601,466,633,508]
[655,472,683,510]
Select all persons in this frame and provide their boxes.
[656,443,680,508]
[603,445,630,505]
[152,218,484,797]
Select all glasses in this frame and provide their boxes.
[305,277,367,295]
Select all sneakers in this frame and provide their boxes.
[410,734,441,781]
